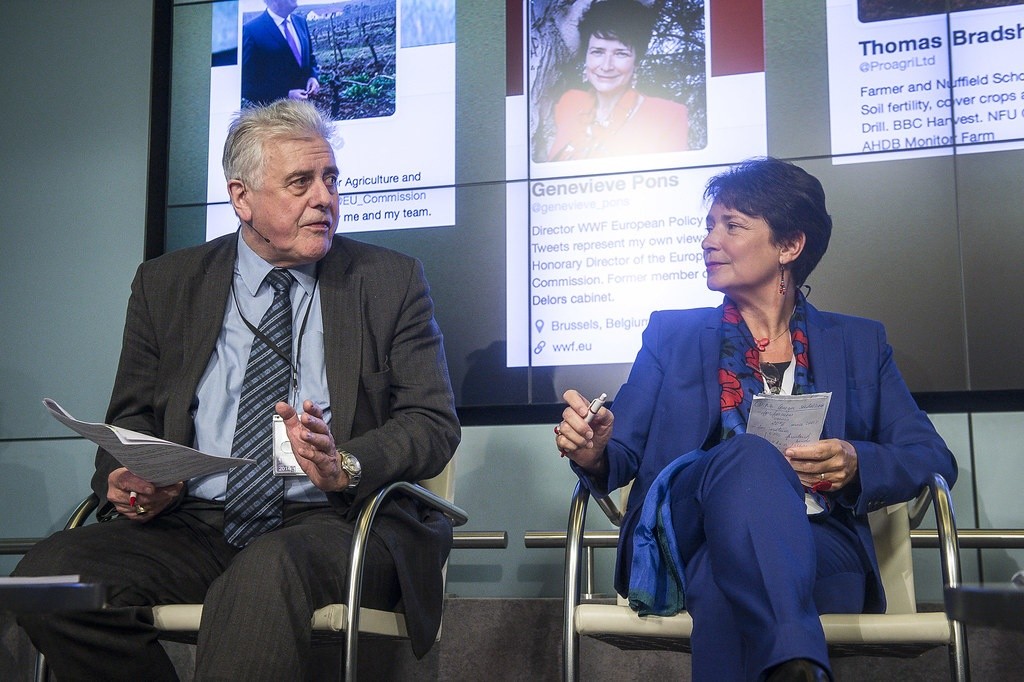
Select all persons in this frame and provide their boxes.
[9,98,462,682]
[549,0,688,161]
[242,0,320,110]
[554,153,960,682]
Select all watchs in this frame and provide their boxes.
[335,448,362,494]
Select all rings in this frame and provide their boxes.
[554,426,561,436]
[821,473,824,482]
[137,505,147,516]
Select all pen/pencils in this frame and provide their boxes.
[129,491,138,509]
[560,391,607,458]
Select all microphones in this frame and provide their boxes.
[245,221,270,243]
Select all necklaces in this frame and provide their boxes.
[753,329,789,352]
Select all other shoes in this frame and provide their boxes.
[766,659,832,682]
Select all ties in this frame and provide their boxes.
[281,19,301,66]
[224,267,296,549]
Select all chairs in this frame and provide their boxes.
[33,430,470,682]
[558,472,970,682]
